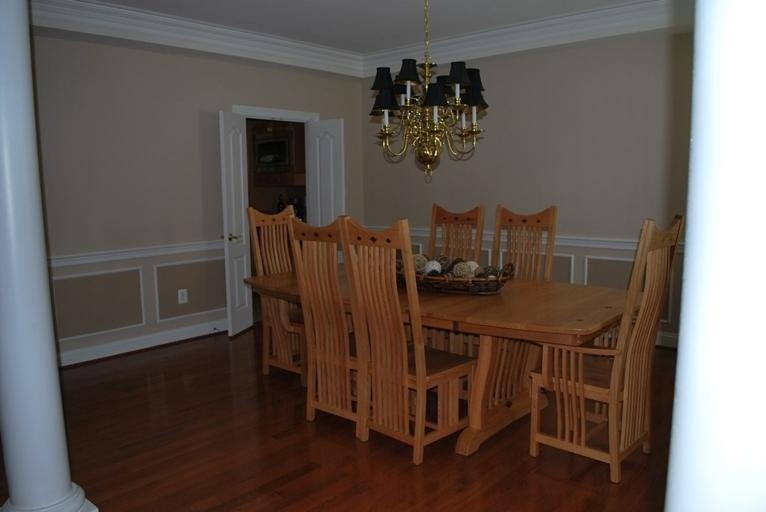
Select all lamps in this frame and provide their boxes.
[370,0,488,180]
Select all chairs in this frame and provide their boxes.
[490,204,557,281]
[338,216,477,463]
[288,216,366,440]
[247,206,310,388]
[528,217,682,478]
[427,205,484,269]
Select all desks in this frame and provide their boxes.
[249,269,645,456]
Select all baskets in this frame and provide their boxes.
[396,263,514,296]
